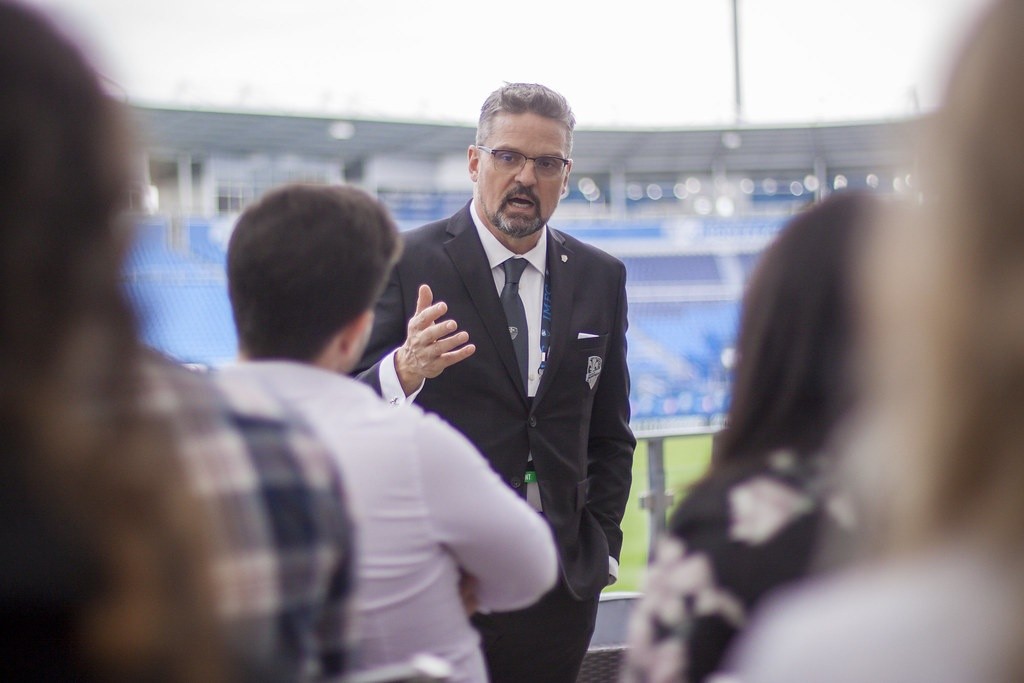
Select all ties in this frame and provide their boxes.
[498,258,529,398]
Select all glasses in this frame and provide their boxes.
[475,145,569,181]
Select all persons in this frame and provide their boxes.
[615,184,930,681]
[1,0,361,681]
[711,0,1024,683]
[181,182,560,683]
[335,85,639,681]
